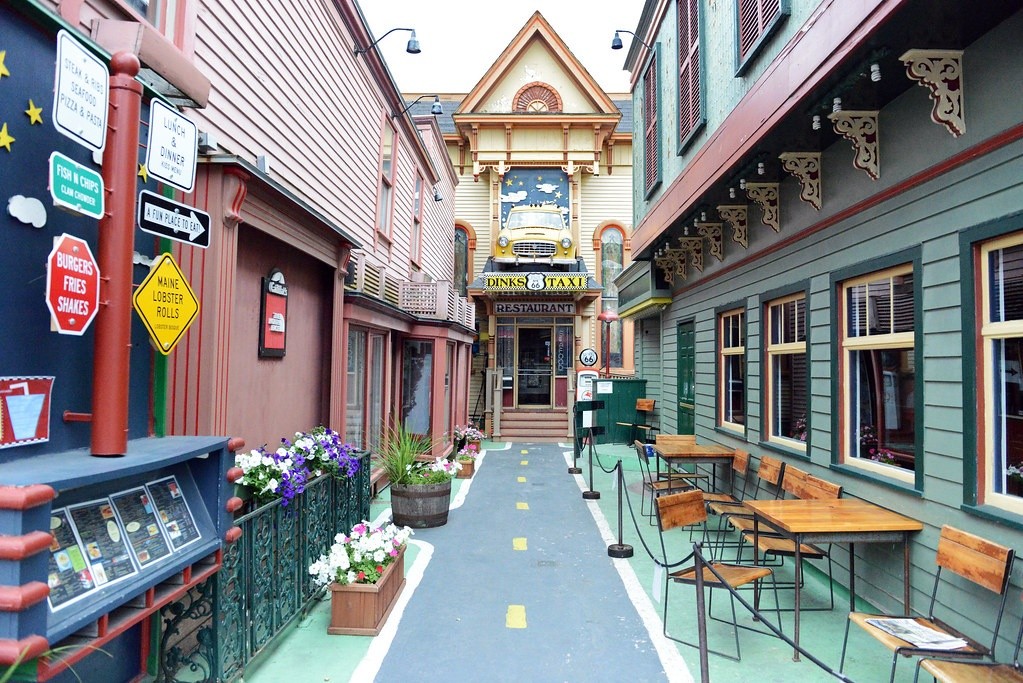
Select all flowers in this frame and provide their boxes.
[860,423,897,467]
[1007,460,1023,483]
[305,518,415,591]
[364,412,463,488]
[229,424,363,507]
[455,420,487,441]
[792,412,808,441]
[455,444,477,462]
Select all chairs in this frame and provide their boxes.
[634,439,702,527]
[733,476,843,611]
[699,456,783,561]
[839,522,1016,683]
[912,616,1022,683]
[653,488,783,662]
[612,398,657,447]
[720,463,811,583]
[682,448,751,543]
[654,434,711,492]
[635,425,659,450]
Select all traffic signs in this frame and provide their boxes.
[44,30,210,358]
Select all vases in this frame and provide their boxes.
[468,439,481,454]
[390,477,451,528]
[457,460,475,479]
[329,545,406,637]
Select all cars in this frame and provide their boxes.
[493,206,577,272]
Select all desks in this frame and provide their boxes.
[652,444,736,494]
[743,500,924,660]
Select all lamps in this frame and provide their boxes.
[353,29,421,58]
[392,95,444,120]
[612,29,653,52]
[433,187,444,201]
